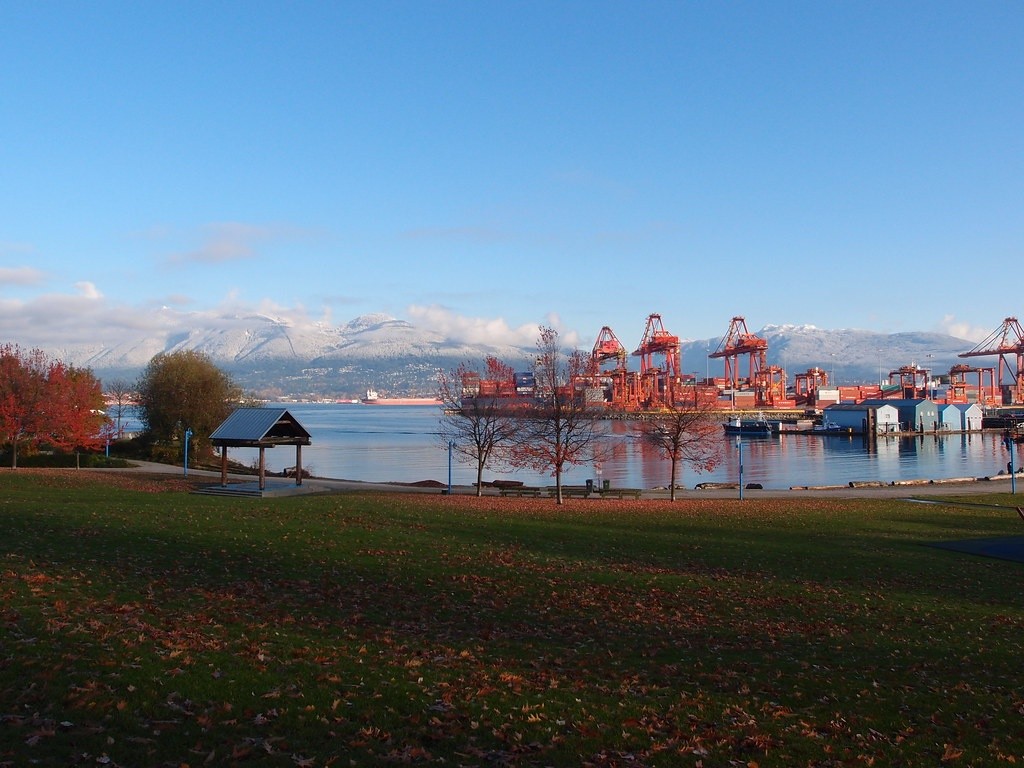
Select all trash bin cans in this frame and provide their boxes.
[603,480,610,489]
[586,479,593,493]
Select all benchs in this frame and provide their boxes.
[598,488,642,500]
[499,485,541,497]
[547,485,591,498]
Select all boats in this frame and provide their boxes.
[812,415,842,432]
[917,374,938,398]
[361,390,444,404]
[724,418,781,435]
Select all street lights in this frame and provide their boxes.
[879,349,883,390]
[736,443,743,500]
[783,348,788,399]
[449,440,458,495]
[706,348,710,386]
[830,354,835,388]
[185,428,194,476]
[1002,439,1016,495]
[927,354,935,401]
